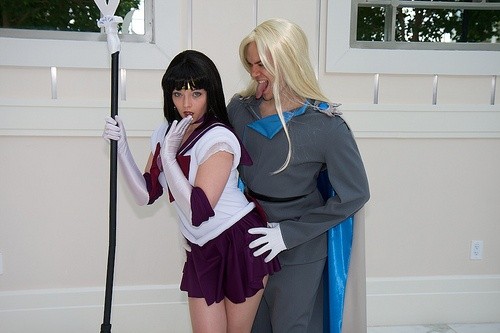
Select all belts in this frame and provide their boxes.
[246,186,313,203]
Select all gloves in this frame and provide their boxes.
[161,114,216,228]
[103,115,163,206]
[247,222,286,263]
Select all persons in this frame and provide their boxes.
[104,50,281,333]
[181,18,371,333]
